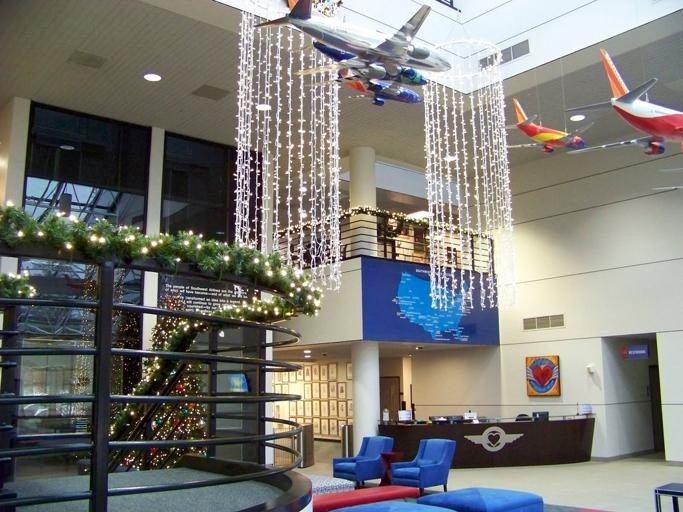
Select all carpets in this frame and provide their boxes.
[296,473,615,512]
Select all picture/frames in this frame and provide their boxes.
[271,360,354,438]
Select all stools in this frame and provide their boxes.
[312,484,545,512]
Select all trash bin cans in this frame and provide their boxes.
[341,424,353,458]
[291,424,314,468]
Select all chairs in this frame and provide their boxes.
[331,434,457,497]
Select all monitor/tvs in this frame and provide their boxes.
[398,409,412,422]
[532,411,549,421]
[447,416,463,424]
